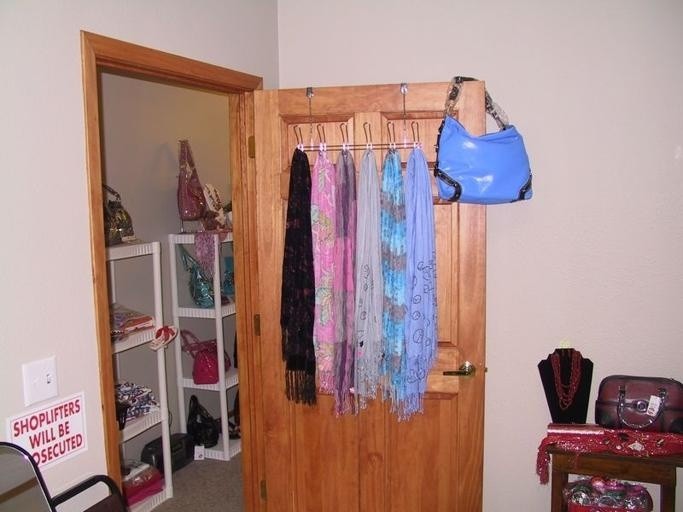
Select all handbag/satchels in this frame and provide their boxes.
[177,140,227,231]
[434,75,533,204]
[595,374,683,432]
[177,244,215,309]
[101,183,134,246]
[180,328,231,384]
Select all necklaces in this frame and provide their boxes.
[551,351,582,410]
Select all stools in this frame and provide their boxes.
[542,430,681,510]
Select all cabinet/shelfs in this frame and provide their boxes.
[103,234,245,510]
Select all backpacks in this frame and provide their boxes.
[187,394,218,448]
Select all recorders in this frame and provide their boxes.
[141,432,195,479]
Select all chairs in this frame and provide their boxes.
[0,441,130,510]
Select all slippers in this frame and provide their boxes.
[148,325,177,351]
